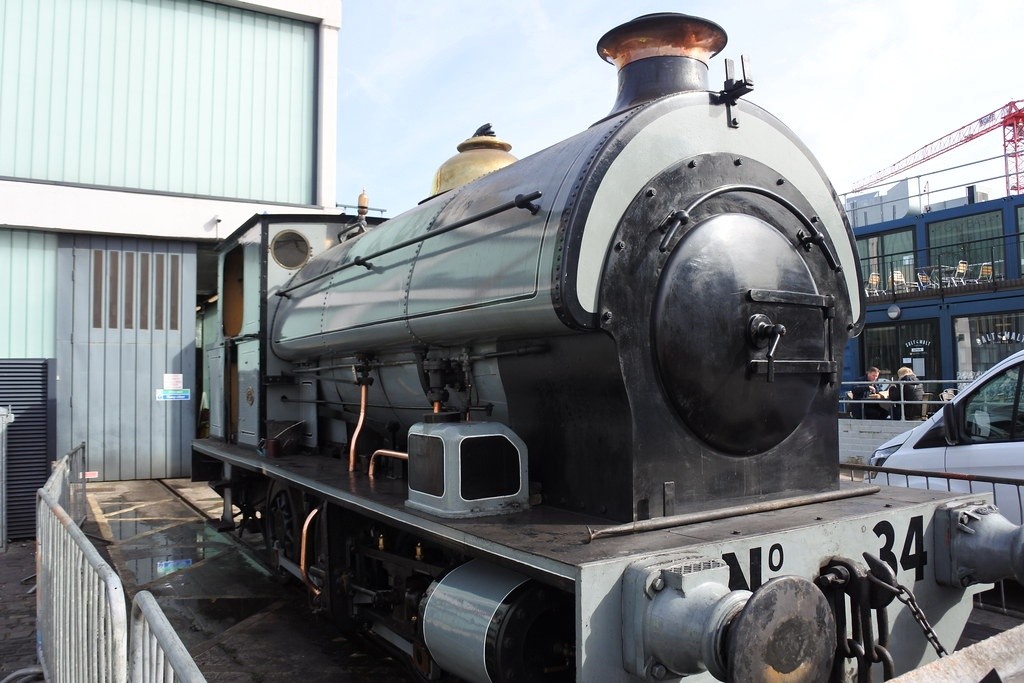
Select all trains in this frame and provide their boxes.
[195,13,1024,683]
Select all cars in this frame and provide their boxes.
[863,349,1024,528]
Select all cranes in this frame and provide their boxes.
[850,99,1023,196]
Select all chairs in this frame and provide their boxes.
[875,272,891,296]
[965,263,994,285]
[939,393,957,402]
[942,260,968,287]
[845,390,854,418]
[918,273,938,290]
[889,271,920,293]
[865,272,881,297]
[944,389,961,396]
[894,392,930,422]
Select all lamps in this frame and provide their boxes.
[920,214,924,219]
[914,215,919,221]
[1001,197,1007,202]
[1009,195,1014,200]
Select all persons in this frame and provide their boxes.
[888,367,924,420]
[851,366,889,420]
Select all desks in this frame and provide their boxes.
[915,265,970,288]
